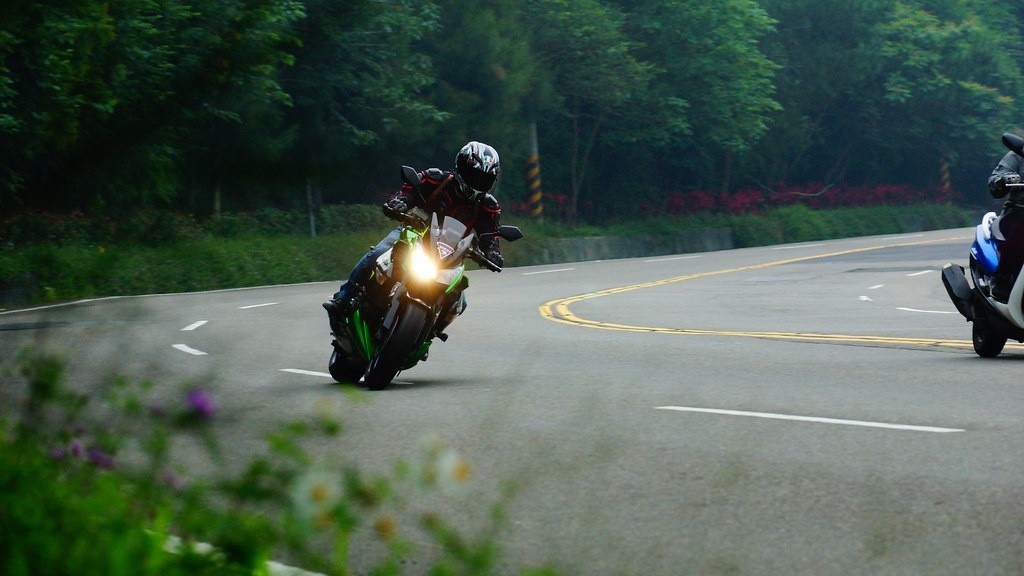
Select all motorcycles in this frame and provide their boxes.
[941,133,1024,358]
[322,165,524,393]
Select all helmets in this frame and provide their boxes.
[453,141,501,202]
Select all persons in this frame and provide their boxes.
[323,140,504,349]
[983,149,1024,302]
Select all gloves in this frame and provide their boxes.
[479,250,505,274]
[383,199,408,222]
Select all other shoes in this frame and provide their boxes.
[989,273,1010,301]
[339,281,355,307]
